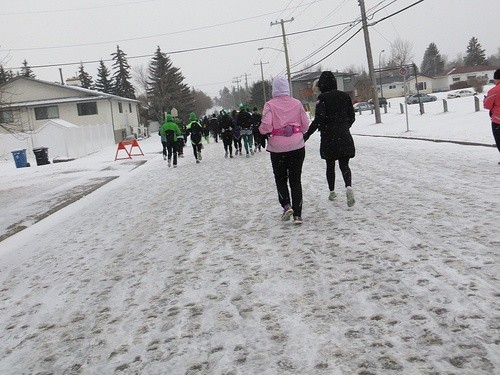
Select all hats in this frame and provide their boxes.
[494,69,500,79]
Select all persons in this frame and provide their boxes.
[216,104,266,158]
[199,114,218,143]
[483,69,500,165]
[303,70,355,207]
[158,114,187,168]
[185,111,203,163]
[259,75,310,223]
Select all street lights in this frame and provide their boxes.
[258,47,294,97]
[379,50,385,106]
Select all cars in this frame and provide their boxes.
[367,97,388,107]
[406,92,437,105]
[447,89,478,100]
[353,102,372,112]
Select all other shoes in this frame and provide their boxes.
[246,149,254,158]
[329,192,338,201]
[346,187,355,207]
[225,152,233,158]
[178,154,185,158]
[168,164,178,168]
[164,154,167,160]
[196,156,202,163]
[235,150,242,156]
[254,148,261,153]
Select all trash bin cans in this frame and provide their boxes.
[10,148,30,168]
[32,146,50,166]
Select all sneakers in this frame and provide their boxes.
[281,205,294,221]
[293,216,304,224]
